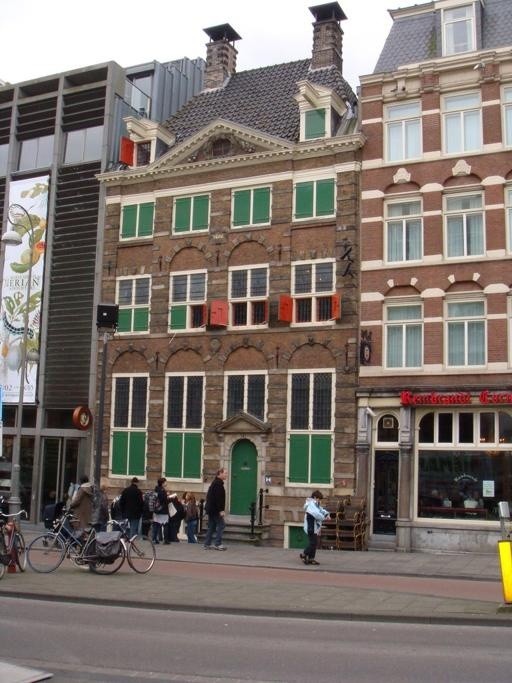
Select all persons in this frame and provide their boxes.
[464,489,479,516]
[66,470,200,549]
[204,469,228,550]
[300,490,332,566]
[475,490,485,505]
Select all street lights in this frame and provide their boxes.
[0,202,34,529]
[84,302,119,573]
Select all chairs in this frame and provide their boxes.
[319,495,370,552]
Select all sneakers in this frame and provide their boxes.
[297,553,320,567]
[202,545,227,552]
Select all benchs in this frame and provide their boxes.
[418,503,488,519]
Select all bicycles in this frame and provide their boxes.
[0,510,27,581]
[27,506,157,576]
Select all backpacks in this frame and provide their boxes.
[147,491,164,514]
